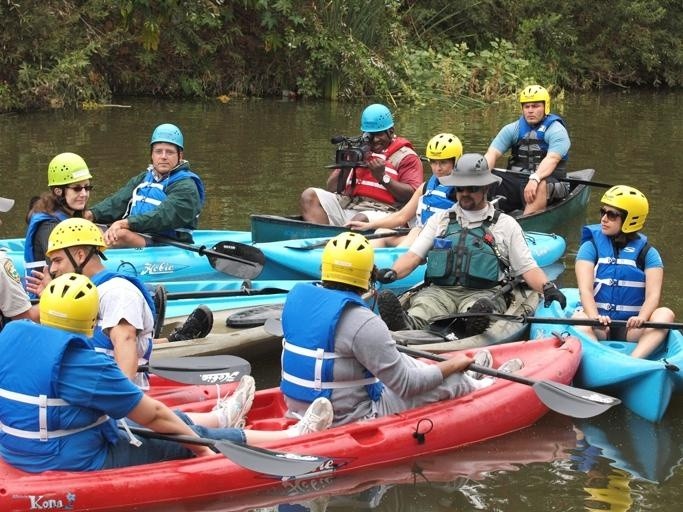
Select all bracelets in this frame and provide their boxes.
[542,283,557,291]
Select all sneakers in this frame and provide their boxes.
[291,396,334,438]
[153,284,167,338]
[480,358,525,383]
[463,348,494,381]
[211,375,256,430]
[464,298,495,339]
[377,290,415,332]
[167,304,213,342]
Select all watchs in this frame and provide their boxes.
[529,173,540,184]
[379,174,391,185]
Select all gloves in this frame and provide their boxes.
[543,281,566,310]
[372,265,396,283]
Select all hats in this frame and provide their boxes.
[0,197,15,213]
[438,153,503,188]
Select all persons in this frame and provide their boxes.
[570,184,675,358]
[299,103,424,230]
[27,123,205,248]
[342,133,463,248]
[25,152,213,344]
[279,231,524,428]
[483,85,572,216]
[24,217,157,391]
[0,273,334,474]
[0,196,40,324]
[376,153,566,339]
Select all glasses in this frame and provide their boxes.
[600,207,624,222]
[65,186,91,192]
[456,186,485,193]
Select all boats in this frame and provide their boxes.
[529,288,683,425]
[0,332,582,512]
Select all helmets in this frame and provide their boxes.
[321,231,376,291]
[150,122,184,151]
[48,151,93,186]
[520,84,551,115]
[39,272,101,340]
[44,217,108,258]
[360,103,395,133]
[601,185,649,234]
[425,132,463,166]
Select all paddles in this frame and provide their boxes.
[118,425,330,476]
[106,223,265,278]
[419,158,614,189]
[430,312,683,330]
[134,355,252,385]
[283,228,409,248]
[395,343,622,418]
